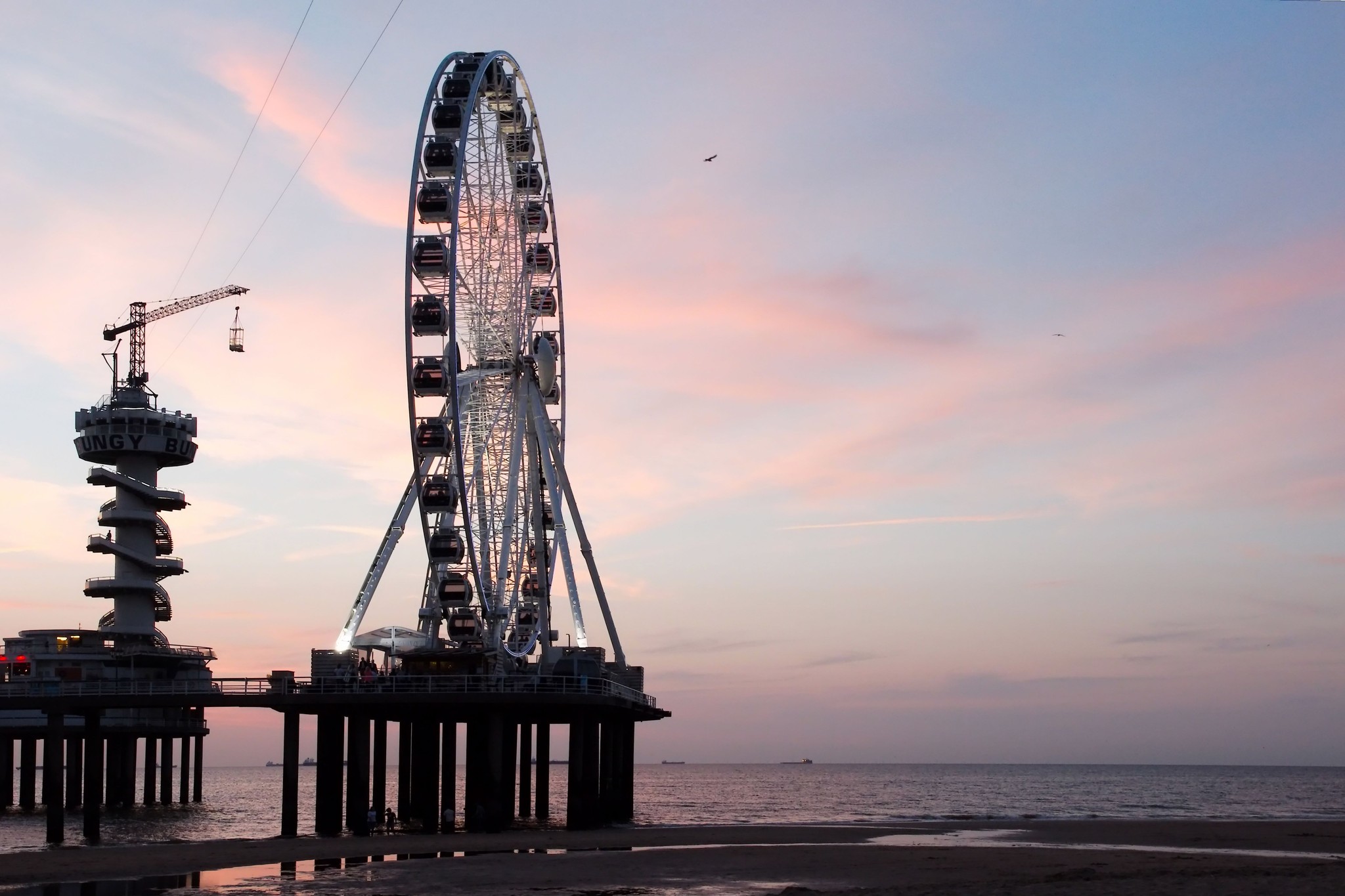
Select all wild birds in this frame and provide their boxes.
[705,154,718,162]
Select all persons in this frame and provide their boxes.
[468,645,476,648]
[333,658,402,693]
[513,584,537,651]
[366,806,376,837]
[443,807,454,834]
[384,807,397,836]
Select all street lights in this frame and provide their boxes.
[566,634,570,647]
[614,653,616,663]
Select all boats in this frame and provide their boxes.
[662,760,685,764]
[302,758,317,766]
[780,758,813,764]
[266,761,284,766]
[156,763,177,768]
[16,765,67,770]
[531,757,569,765]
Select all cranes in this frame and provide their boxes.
[102,284,250,393]
